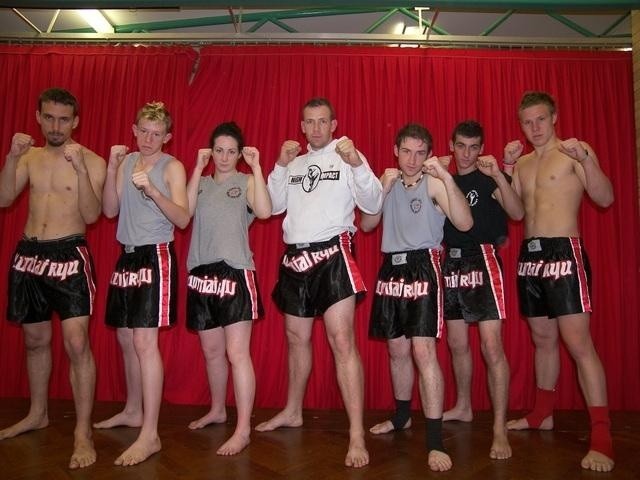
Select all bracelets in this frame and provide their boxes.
[503,161,518,168]
[577,149,589,163]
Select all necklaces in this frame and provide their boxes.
[400,170,424,188]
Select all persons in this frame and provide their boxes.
[186,121,272,456]
[360,125,475,473]
[504,93,615,472]
[93,101,191,467]
[440,119,524,461]
[254,96,383,469]
[0,89,106,469]
